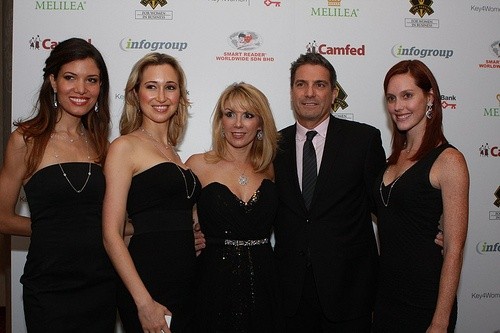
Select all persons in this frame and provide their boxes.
[195,53,444,333]
[0,37,135,333]
[372,60,470,333]
[184,80,279,333]
[100,50,202,333]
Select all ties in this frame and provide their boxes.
[301,131,318,213]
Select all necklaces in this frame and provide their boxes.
[227,155,251,186]
[140,126,197,200]
[48,123,92,193]
[379,156,427,207]
